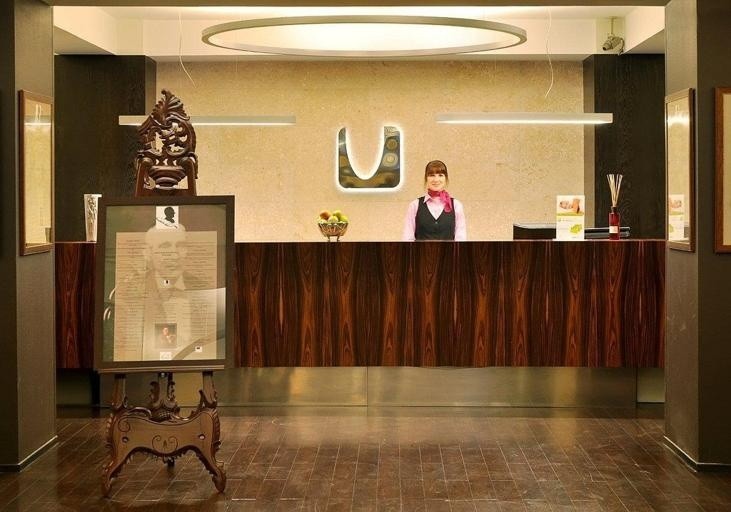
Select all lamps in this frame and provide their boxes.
[433,6,612,125]
[119,8,296,129]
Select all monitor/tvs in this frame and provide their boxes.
[513,223,556,239]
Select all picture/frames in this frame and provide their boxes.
[19,88,54,257]
[664,88,729,255]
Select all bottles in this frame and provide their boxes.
[608,206,623,240]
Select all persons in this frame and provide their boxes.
[125,206,213,349]
[404,160,468,241]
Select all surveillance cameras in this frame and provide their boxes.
[602,36,621,51]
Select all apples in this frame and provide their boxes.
[319,211,348,223]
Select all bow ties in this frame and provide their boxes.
[159,287,184,302]
